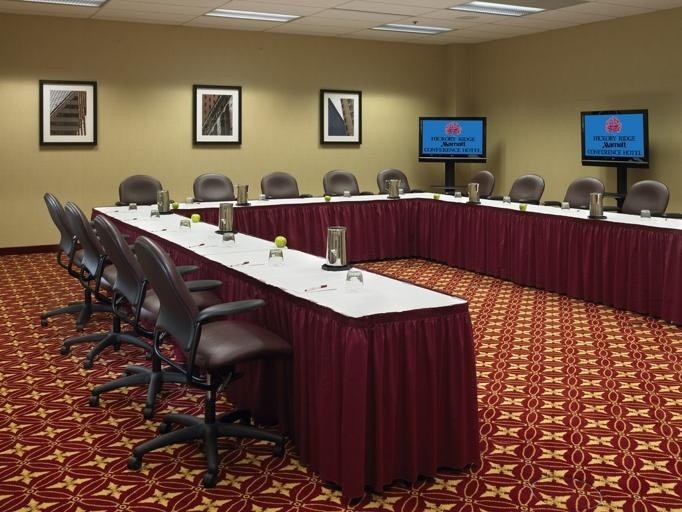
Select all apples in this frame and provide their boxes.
[518,203,527,211]
[172,200,179,209]
[433,194,440,200]
[325,195,331,202]
[275,235,287,248]
[191,213,201,223]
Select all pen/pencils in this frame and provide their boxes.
[305,285,327,291]
[231,261,249,268]
[122,217,139,222]
[152,229,167,232]
[189,244,205,248]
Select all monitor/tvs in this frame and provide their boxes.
[581,109,650,170]
[418,116,487,164]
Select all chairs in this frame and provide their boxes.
[261,171,313,200]
[116,174,177,207]
[602,178,682,220]
[59,199,159,369]
[445,170,495,200]
[488,173,546,205]
[87,215,223,421]
[42,192,125,331]
[376,169,427,194]
[543,176,605,208]
[193,171,238,204]
[320,169,375,197]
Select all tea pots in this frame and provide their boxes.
[157,189,169,212]
[326,225,348,266]
[467,183,481,203]
[384,178,401,198]
[219,203,233,231]
[589,192,604,217]
[232,184,249,203]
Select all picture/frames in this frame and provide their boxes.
[320,89,362,145]
[39,80,97,145]
[193,84,241,145]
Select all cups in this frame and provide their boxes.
[455,192,461,199]
[259,194,265,200]
[640,210,651,221]
[503,196,510,206]
[185,198,193,205]
[268,249,285,268]
[561,202,570,212]
[343,191,350,198]
[345,271,364,292]
[151,209,160,220]
[129,203,137,213]
[223,233,235,248]
[178,219,191,234]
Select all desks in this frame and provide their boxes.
[91,192,682,502]
